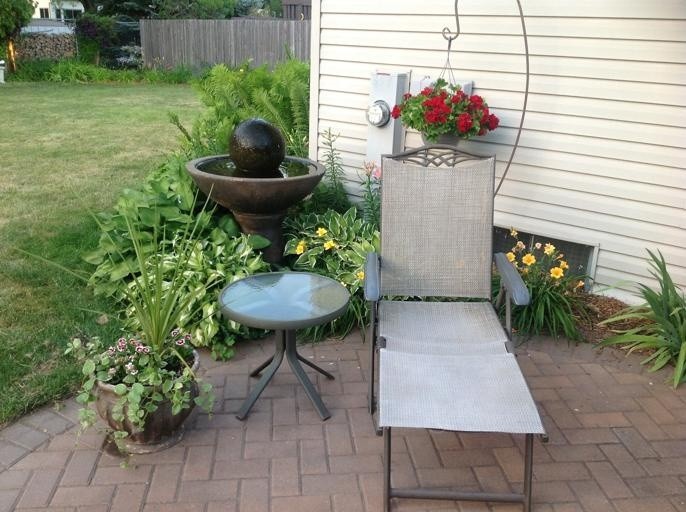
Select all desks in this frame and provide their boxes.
[217,270,354,428]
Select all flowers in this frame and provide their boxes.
[389,79,499,144]
[13,179,235,472]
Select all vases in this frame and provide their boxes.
[421,130,461,148]
[87,345,201,454]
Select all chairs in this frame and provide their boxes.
[363,141,550,512]
